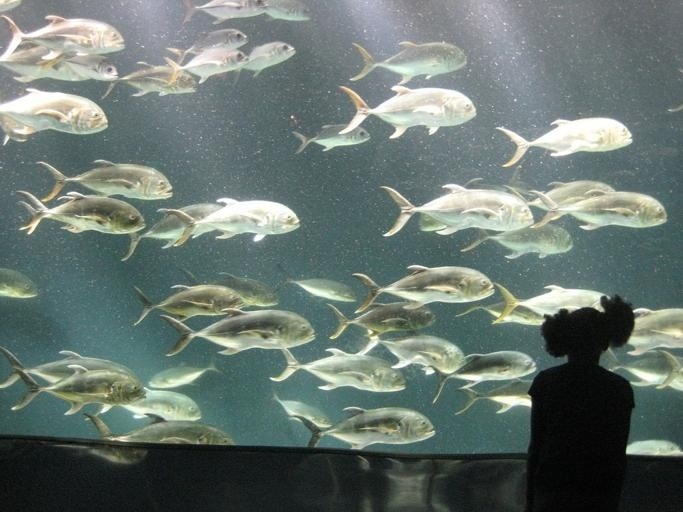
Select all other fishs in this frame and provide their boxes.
[0,0,683,457]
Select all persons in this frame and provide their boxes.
[525,294,634,512]
